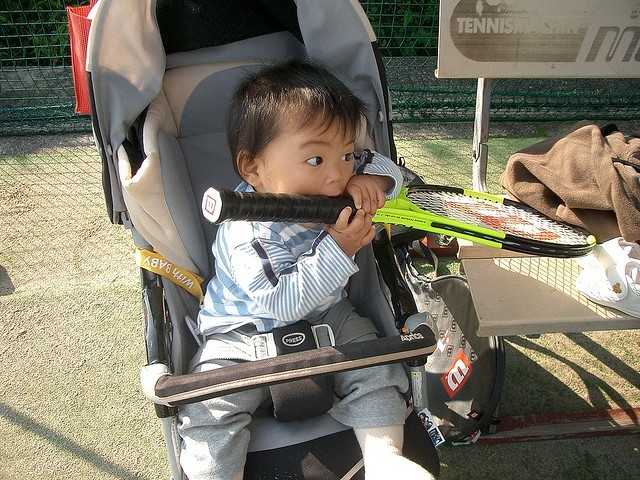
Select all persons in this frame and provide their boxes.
[175,55,434,479]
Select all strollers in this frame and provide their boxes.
[65,2,460,480]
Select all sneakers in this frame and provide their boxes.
[576,260,640,318]
[575,237,640,268]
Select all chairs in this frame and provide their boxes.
[435,0,640,443]
